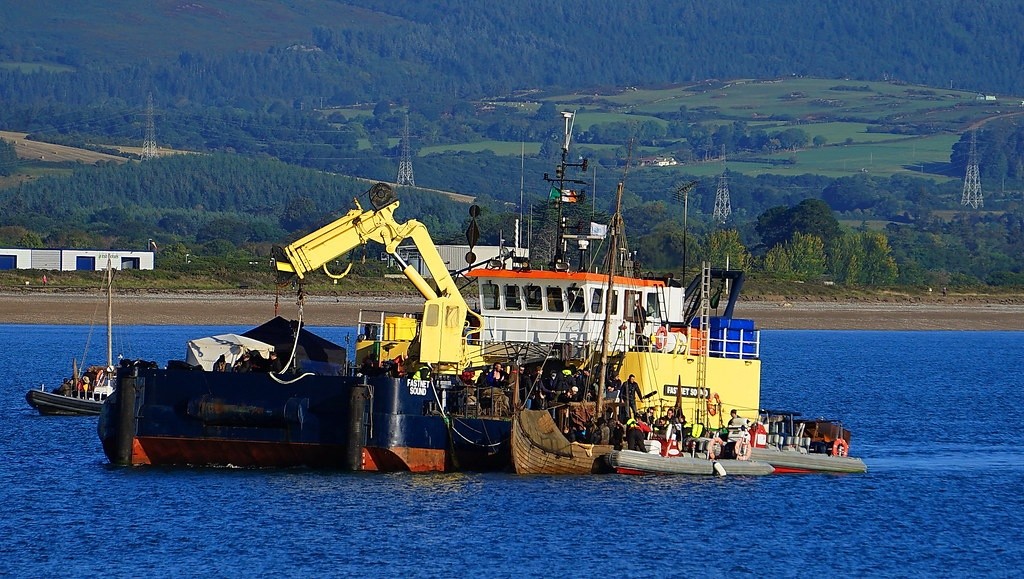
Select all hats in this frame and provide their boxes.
[83,376,90,383]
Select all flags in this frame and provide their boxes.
[548,186,577,203]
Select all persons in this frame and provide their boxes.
[564,406,750,453]
[214,352,283,374]
[633,300,646,345]
[292,279,296,290]
[943,286,947,297]
[59,365,106,399]
[461,361,645,420]
[389,354,432,415]
[42,275,46,286]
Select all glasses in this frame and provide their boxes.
[650,412,653,414]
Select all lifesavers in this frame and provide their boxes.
[707,392,721,416]
[655,325,668,349]
[734,438,752,461]
[832,438,849,457]
[95,370,106,385]
[708,436,725,459]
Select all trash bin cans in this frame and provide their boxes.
[365,323,378,340]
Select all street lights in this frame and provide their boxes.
[271,258,276,275]
[249,262,258,285]
[185,254,189,263]
[148,239,152,250]
[335,261,338,266]
[269,261,272,266]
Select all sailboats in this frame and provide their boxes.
[509,182,684,476]
[25,257,119,415]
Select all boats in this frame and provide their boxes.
[94,110,762,474]
[715,411,867,475]
[601,446,775,476]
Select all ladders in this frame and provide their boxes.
[697,260,711,426]
